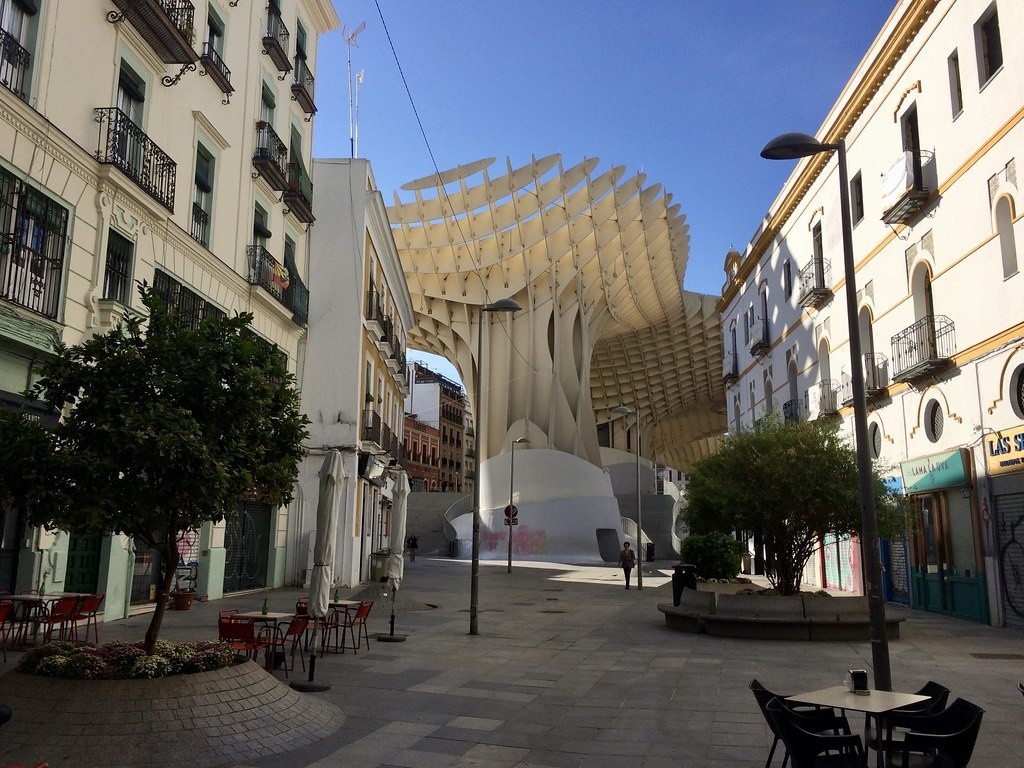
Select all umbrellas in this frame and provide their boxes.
[306,449,346,682]
[386,471,411,636]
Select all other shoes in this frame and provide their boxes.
[625,586,630,589]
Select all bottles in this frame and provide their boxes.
[334,588,339,602]
[262,599,268,614]
[40,582,46,596]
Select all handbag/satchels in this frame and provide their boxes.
[633,562,635,568]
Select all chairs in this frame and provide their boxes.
[748,679,986,768]
[218,597,374,678]
[0,590,105,663]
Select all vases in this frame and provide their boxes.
[172,592,196,610]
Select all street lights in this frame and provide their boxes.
[613,406,643,589]
[760,133,896,728]
[468,299,524,635]
[507,437,531,573]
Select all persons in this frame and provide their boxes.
[618,542,635,589]
[407,534,418,562]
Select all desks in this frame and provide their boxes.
[328,600,361,653]
[0,593,91,645]
[784,686,932,768]
[233,611,296,673]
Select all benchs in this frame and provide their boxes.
[665,590,906,641]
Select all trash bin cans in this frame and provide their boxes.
[671,562,697,606]
[647,542,655,562]
[370,547,390,580]
